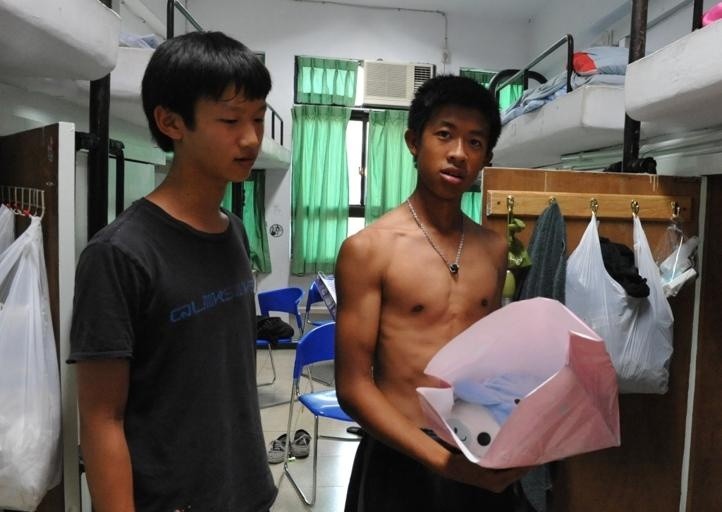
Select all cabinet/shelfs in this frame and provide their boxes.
[1,120,81,512]
[482,166,721,511]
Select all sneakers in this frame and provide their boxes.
[292,429,312,457]
[268,433,289,463]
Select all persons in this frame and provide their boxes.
[67,30,273,512]
[335,74,546,512]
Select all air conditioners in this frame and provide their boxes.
[362,61,435,107]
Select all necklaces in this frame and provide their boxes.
[404,194,469,274]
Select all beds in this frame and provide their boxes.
[489,0,722,174]
[0,3,293,169]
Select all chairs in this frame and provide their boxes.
[258,278,363,509]
